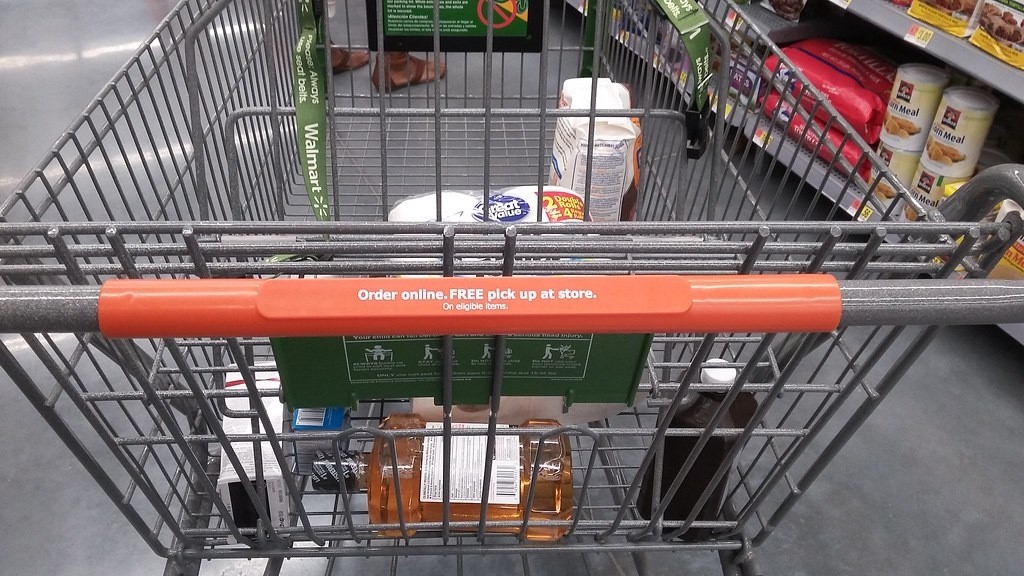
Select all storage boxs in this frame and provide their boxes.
[727,48,769,111]
[906,0,985,39]
[291,406,352,476]
[702,0,831,45]
[936,179,1004,271]
[968,0,1024,71]
[216,361,303,544]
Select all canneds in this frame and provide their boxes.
[866,64,998,228]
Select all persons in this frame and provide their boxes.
[330,38,446,92]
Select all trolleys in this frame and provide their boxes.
[1,1,1023,575]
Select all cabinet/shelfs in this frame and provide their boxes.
[566,0,1024,348]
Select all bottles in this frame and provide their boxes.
[313,413,573,540]
[636,359,758,540]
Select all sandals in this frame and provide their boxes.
[331,49,369,73]
[372,55,445,93]
